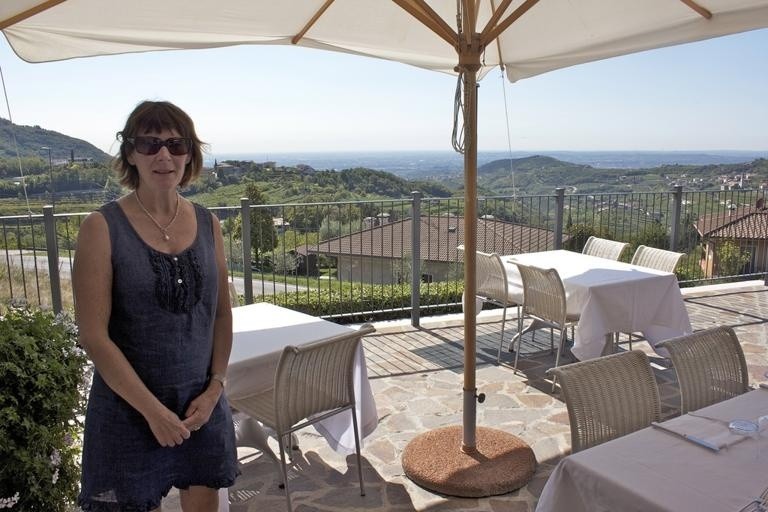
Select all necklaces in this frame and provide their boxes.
[135,189,180,240]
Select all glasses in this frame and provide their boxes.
[125,135,194,156]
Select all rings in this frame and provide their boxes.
[193,426,200,431]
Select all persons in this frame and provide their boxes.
[77,100,245,510]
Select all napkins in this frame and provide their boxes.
[651,412,747,449]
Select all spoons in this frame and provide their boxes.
[687,410,759,435]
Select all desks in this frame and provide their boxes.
[498,251,692,362]
[218,301,362,512]
[561,387,767,512]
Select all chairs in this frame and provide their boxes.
[655,326,750,415]
[456,244,537,363]
[545,349,666,454]
[507,260,582,392]
[566,236,631,341]
[226,322,375,512]
[615,244,689,351]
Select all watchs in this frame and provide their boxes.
[212,374,227,389]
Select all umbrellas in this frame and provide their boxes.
[0,1,768,452]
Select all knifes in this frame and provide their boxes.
[650,421,720,451]
